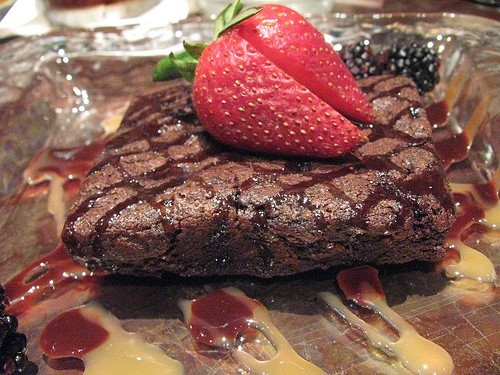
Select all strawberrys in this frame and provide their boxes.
[150,1,375,158]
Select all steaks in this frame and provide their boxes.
[60,73,453,287]
